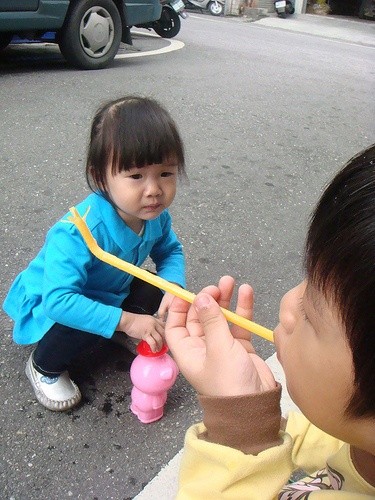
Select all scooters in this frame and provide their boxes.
[132,0,188,38]
[274,0,296,17]
[184,0,225,16]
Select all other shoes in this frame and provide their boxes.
[112,331,142,356]
[24,347,81,411]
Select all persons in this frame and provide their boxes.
[3,95,187,412]
[163,142,375,500]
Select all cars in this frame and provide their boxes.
[0,0,163,69]
